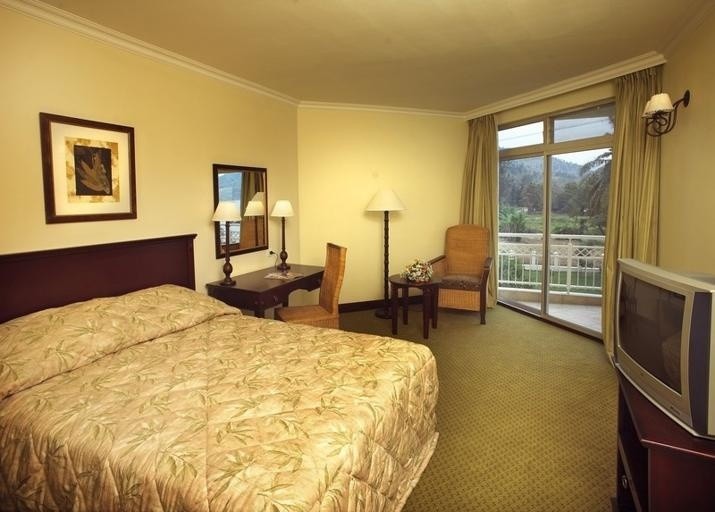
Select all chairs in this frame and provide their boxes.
[272,242,347,328]
[427,224,493,325]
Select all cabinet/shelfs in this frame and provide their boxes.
[610,364,715,511]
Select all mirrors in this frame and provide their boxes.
[212,163,269,259]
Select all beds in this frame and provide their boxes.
[0,232,441,512]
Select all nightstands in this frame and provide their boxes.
[205,263,325,319]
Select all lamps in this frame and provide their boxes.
[641,90,689,136]
[213,202,242,286]
[646,89,690,134]
[363,188,406,319]
[270,200,295,272]
[243,192,266,246]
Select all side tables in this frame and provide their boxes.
[388,272,441,339]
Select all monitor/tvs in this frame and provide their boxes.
[616,257,715,443]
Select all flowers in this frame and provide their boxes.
[400,256,434,282]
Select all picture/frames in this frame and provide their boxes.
[39,111,137,225]
